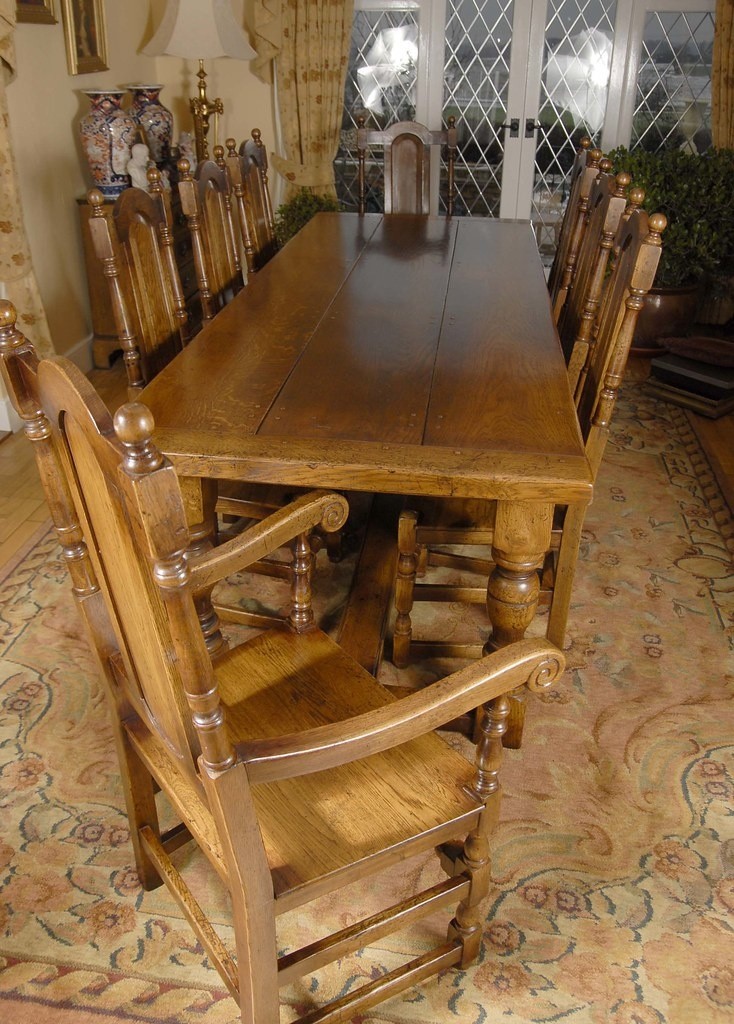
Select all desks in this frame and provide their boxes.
[129,213,596,751]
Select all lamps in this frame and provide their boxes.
[140,0,259,161]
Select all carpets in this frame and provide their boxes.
[0,365,734,1024]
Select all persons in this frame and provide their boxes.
[125,144,153,188]
[176,131,198,173]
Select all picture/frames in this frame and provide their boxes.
[12,0,59,25]
[60,0,113,76]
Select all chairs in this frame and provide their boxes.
[0,114,667,1024]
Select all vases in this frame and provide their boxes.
[124,83,174,169]
[75,88,137,198]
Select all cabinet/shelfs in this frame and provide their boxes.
[76,162,262,370]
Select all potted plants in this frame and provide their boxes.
[601,144,734,360]
